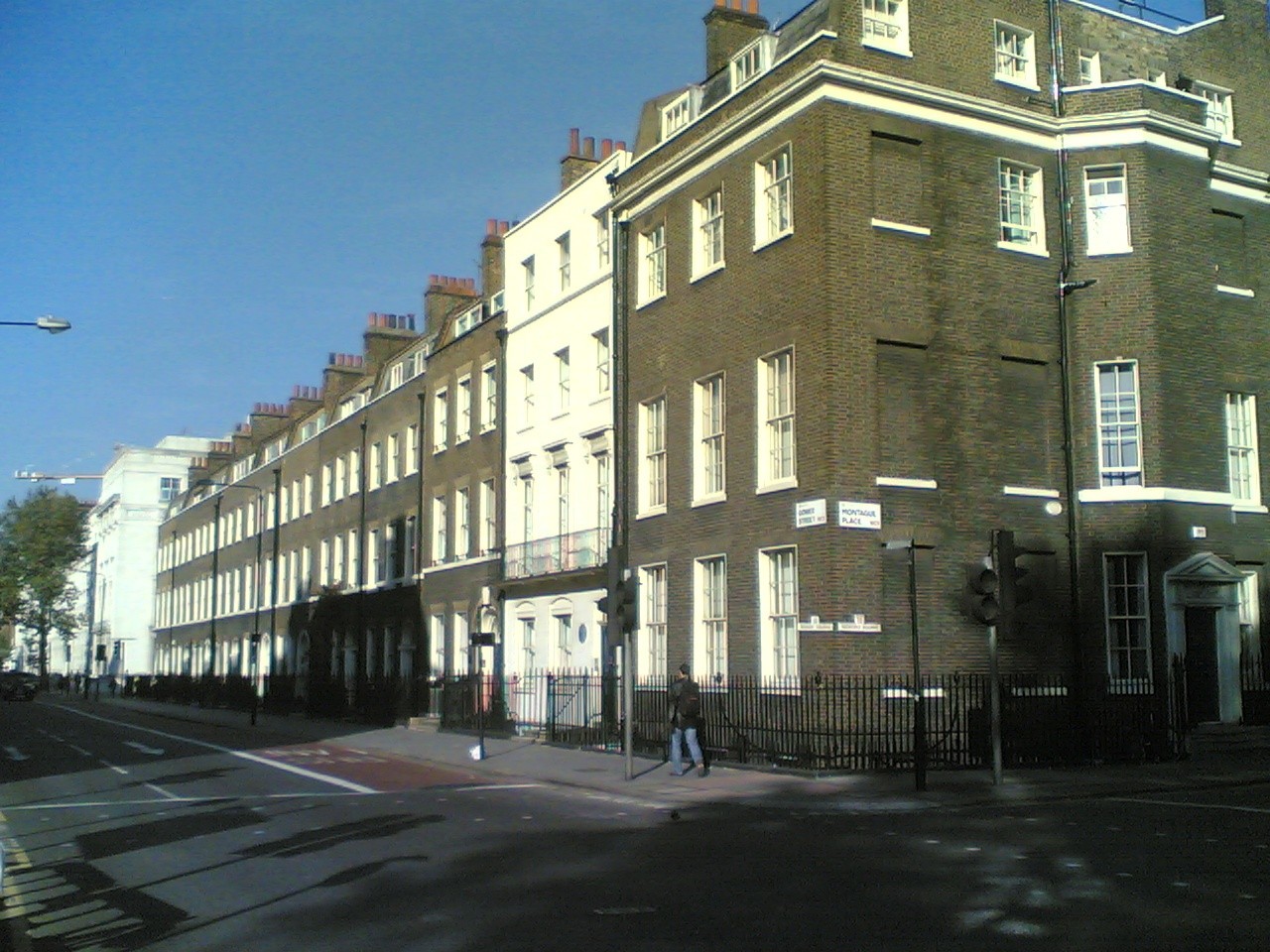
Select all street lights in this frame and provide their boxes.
[196,477,264,725]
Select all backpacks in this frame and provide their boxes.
[678,681,701,718]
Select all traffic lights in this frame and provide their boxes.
[960,561,999,624]
[991,529,1031,617]
[615,577,641,631]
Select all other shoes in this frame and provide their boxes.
[699,764,705,777]
[669,771,683,777]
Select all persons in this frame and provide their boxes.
[668,663,705,777]
[249,695,258,726]
[52,672,119,700]
